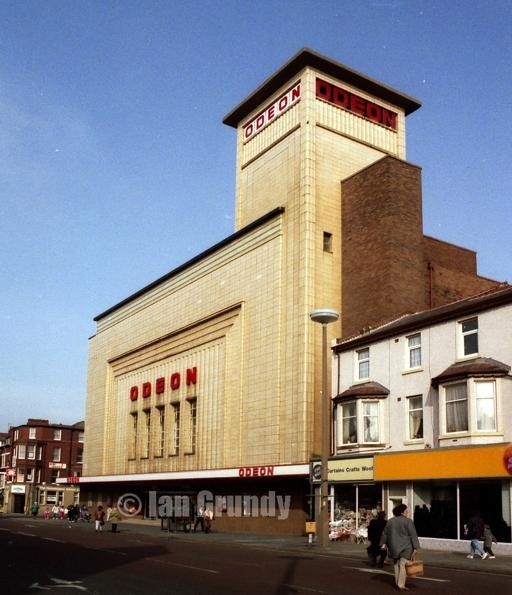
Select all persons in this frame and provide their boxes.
[367,511,389,566]
[379,504,421,591]
[31,502,40,518]
[194,505,215,533]
[44,504,120,533]
[480,522,498,559]
[466,512,487,561]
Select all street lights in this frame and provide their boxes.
[309,310,340,548]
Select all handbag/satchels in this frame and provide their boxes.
[405,550,424,579]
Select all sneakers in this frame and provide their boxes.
[465,552,496,560]
